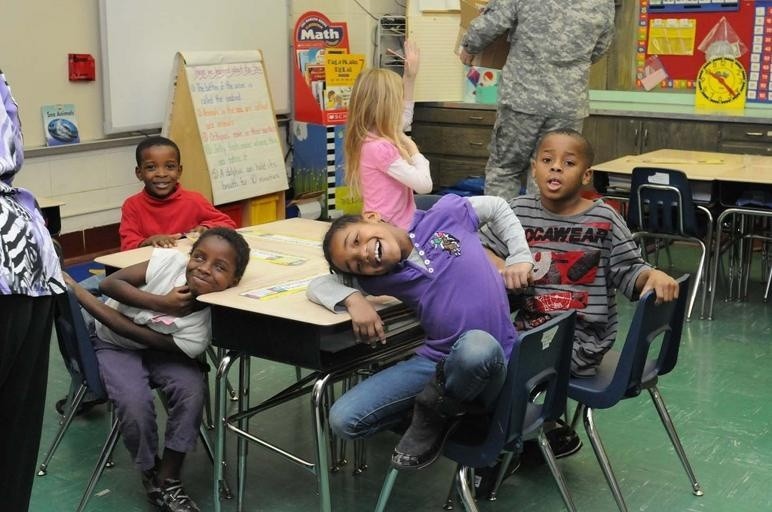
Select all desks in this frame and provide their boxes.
[93,212,434,511]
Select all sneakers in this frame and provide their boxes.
[517,419,585,468]
[139,466,204,512]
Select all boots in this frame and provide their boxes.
[388,365,472,472]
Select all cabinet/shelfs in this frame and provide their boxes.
[409,102,499,192]
[719,124,772,155]
[375,13,407,74]
[582,114,719,186]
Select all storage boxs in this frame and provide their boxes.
[246,196,279,225]
[452,0,514,71]
[213,202,246,229]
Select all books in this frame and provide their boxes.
[40,102,79,147]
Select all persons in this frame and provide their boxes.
[476,125,680,467]
[60,225,260,510]
[304,45,365,117]
[460,0,621,222]
[312,191,538,494]
[0,72,75,512]
[113,136,239,248]
[345,37,439,231]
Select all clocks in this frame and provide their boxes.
[694,57,747,117]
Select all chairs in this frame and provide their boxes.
[625,164,723,324]
[706,177,771,322]
[45,269,232,511]
[375,309,587,512]
[561,273,704,511]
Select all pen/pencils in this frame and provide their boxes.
[387,48,406,59]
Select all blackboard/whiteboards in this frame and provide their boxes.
[98,0,291,135]
